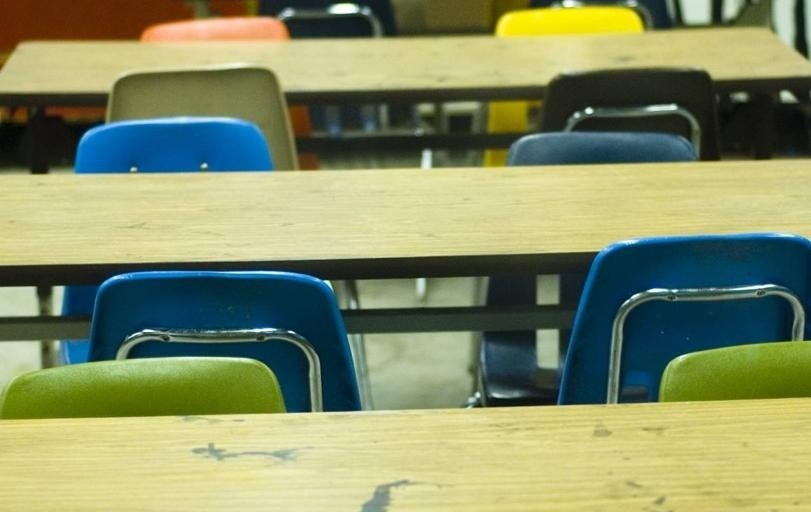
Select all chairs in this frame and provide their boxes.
[2,1,811,415]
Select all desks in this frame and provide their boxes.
[0,397,808,512]
